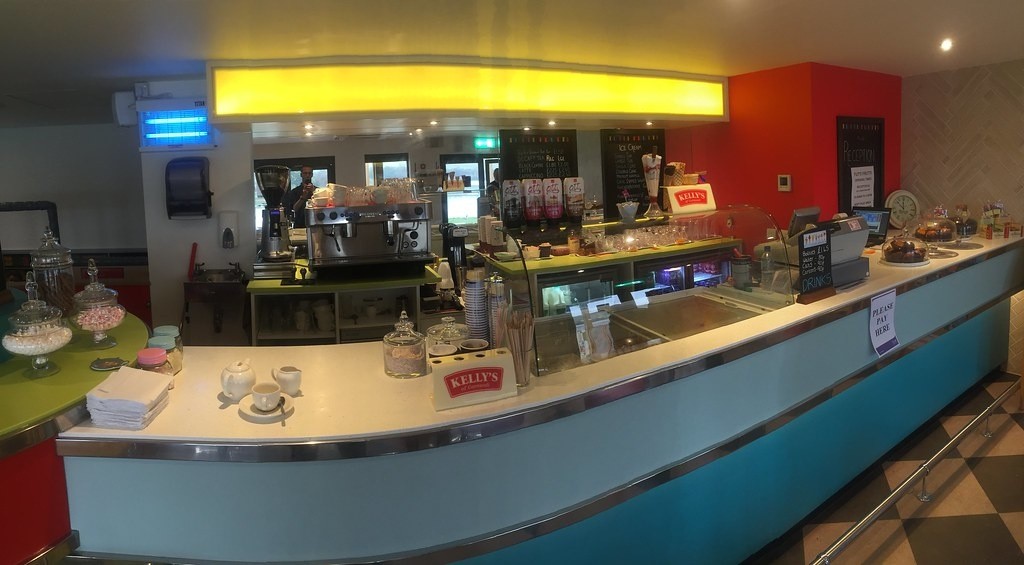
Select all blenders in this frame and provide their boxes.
[255,165,293,261]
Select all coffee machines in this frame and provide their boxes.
[439,223,468,296]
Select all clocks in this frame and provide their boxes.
[885,190,921,229]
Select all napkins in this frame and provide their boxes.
[85,366,172,430]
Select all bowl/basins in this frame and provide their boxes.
[493,252,517,261]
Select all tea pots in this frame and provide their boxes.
[220,357,257,404]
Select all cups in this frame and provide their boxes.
[478,215,503,246]
[261,295,409,337]
[428,344,457,358]
[306,177,419,207]
[730,254,753,290]
[595,216,719,254]
[664,161,707,186]
[459,271,505,351]
[508,344,535,387]
[252,382,281,412]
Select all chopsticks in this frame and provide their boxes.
[506,310,535,384]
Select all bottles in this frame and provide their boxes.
[432,250,454,290]
[427,316,472,348]
[760,246,774,290]
[383,312,427,380]
[135,325,184,390]
[985,222,1010,239]
[567,228,595,253]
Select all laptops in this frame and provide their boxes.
[850,206,892,248]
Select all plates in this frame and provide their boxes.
[238,392,294,418]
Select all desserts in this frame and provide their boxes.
[884,240,925,262]
[914,226,954,242]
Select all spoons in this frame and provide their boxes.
[277,397,286,415]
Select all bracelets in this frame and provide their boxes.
[300,196,306,200]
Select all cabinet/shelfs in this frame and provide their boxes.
[247,257,443,347]
[472,238,744,320]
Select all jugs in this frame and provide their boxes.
[270,365,302,396]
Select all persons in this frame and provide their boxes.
[290,164,319,228]
[489,168,499,189]
